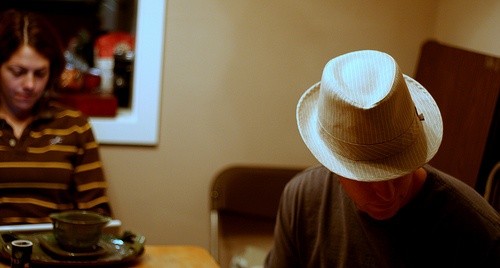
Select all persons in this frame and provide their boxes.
[264,50,497,268]
[0,10,113,225]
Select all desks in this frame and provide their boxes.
[0,245,218,268]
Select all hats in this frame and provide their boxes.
[295,50,443,182]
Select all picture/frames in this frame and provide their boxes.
[0,0,167,146]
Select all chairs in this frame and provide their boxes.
[209,38,500,268]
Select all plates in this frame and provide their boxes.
[1,228,144,265]
[38,234,110,259]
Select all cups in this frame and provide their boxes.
[50,211,111,251]
